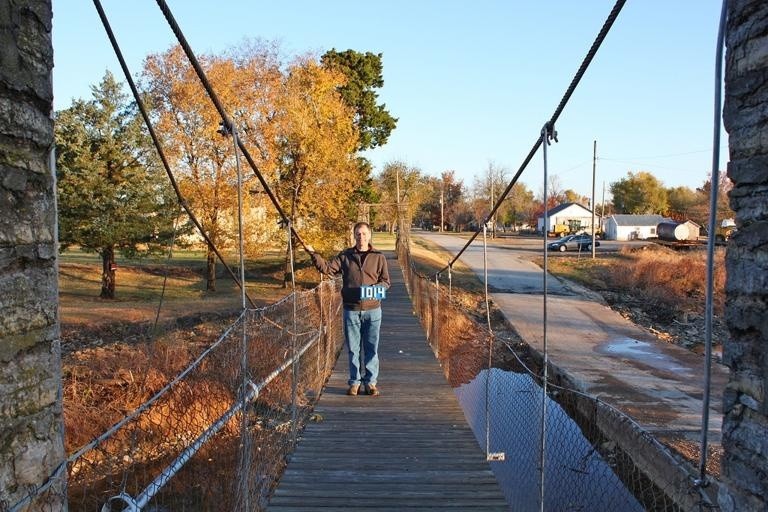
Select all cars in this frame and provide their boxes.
[548,234,600,252]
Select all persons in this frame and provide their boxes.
[305,222,391,396]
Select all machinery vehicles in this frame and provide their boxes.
[552,219,582,237]
[714,219,737,245]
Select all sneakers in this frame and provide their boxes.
[365,383,379,395]
[348,383,360,395]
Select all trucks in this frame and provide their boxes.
[421,219,441,232]
